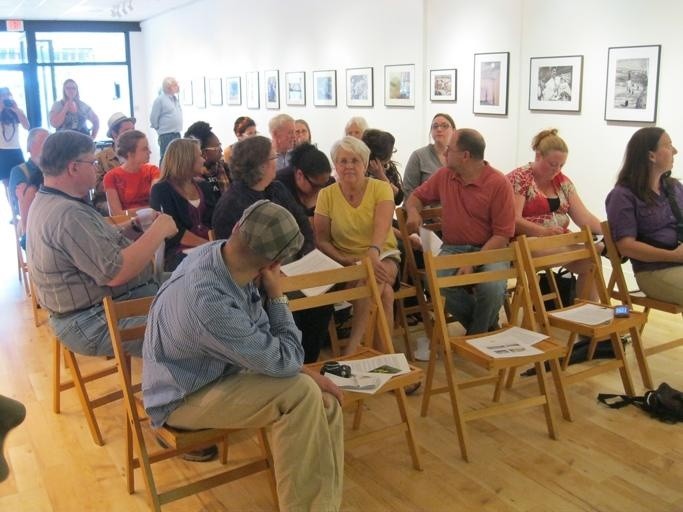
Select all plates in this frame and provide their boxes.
[413,350,437,361]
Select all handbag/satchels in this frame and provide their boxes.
[644,383,683,422]
[534,267,575,311]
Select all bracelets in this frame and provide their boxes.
[132,218,144,233]
[390,180,392,184]
[267,295,289,306]
[368,246,380,256]
[472,265,476,272]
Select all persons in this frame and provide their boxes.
[0,87,31,223]
[149,76,183,171]
[142,200,343,512]
[506,129,605,314]
[406,129,515,336]
[313,133,400,351]
[92,114,368,222]
[10,128,53,251]
[606,127,683,312]
[150,139,220,273]
[403,113,456,236]
[361,128,426,306]
[24,130,218,461]
[543,68,562,101]
[557,77,569,99]
[213,141,334,363]
[49,79,99,139]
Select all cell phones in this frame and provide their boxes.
[614,305,629,318]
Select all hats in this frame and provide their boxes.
[237,200,304,259]
[107,113,136,137]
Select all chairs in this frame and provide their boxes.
[14,204,442,449]
[421,240,559,463]
[492,225,648,423]
[278,255,426,475]
[102,294,281,511]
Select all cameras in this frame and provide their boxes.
[320,361,351,378]
[3,99,13,107]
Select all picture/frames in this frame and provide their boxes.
[346,67,374,107]
[472,52,510,116]
[528,55,583,112]
[312,70,337,106]
[264,70,280,110]
[226,76,242,105]
[604,46,660,122]
[384,64,415,107]
[284,72,306,106]
[246,71,260,110]
[430,69,457,101]
[180,75,223,109]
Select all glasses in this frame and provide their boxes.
[205,143,221,151]
[306,176,331,188]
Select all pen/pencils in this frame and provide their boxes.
[160,205,164,213]
[551,211,558,225]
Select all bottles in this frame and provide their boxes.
[69,100,76,113]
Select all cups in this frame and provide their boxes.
[136,208,159,234]
[416,338,429,356]
